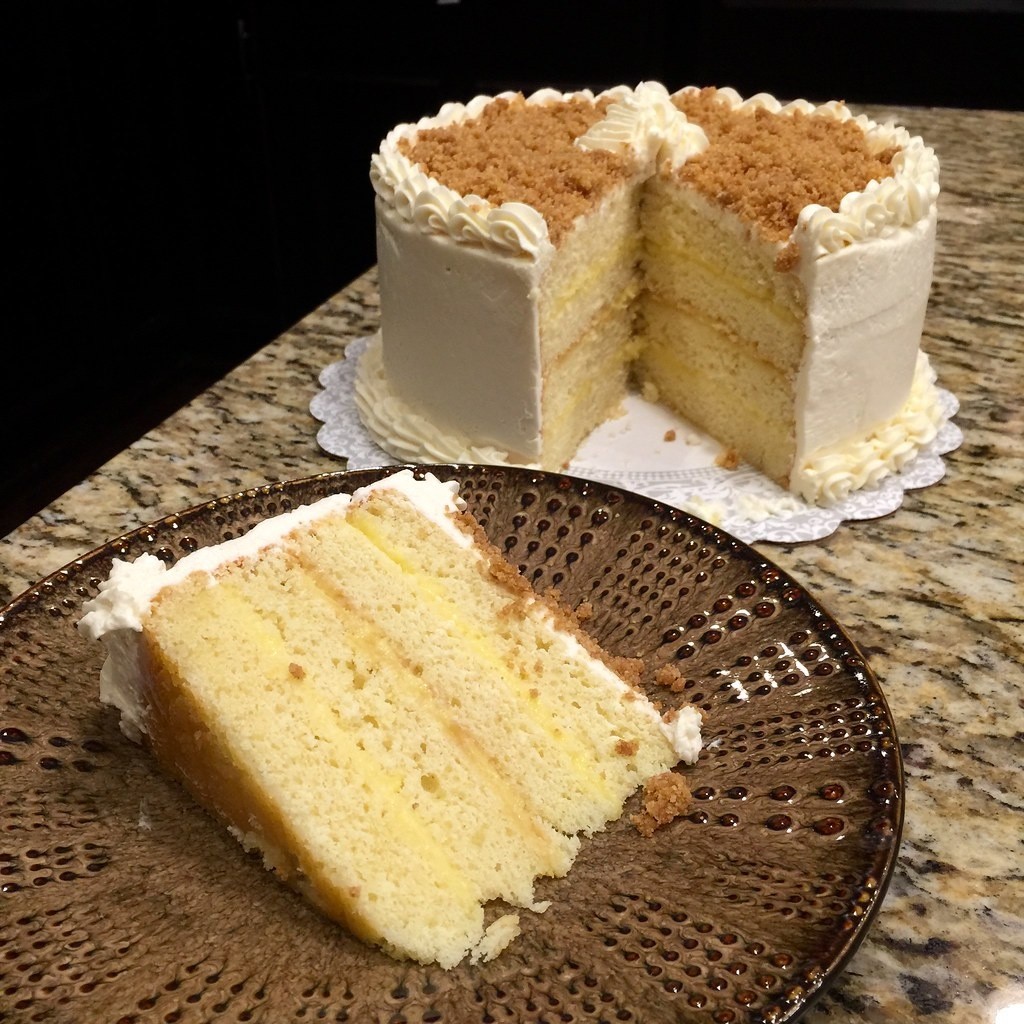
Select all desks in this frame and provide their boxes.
[0,102,1024,1024]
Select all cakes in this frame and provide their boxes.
[77,469,704,970]
[352,79,951,506]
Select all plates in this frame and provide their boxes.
[0,464,902,1024]
